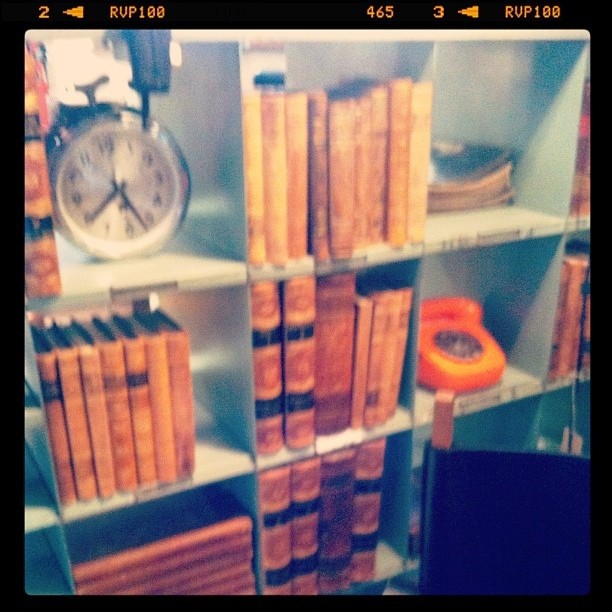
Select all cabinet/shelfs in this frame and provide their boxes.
[26,30,589,596]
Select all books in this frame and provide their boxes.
[132,308,195,482]
[48,325,97,502]
[261,88,286,267]
[313,272,357,435]
[257,463,291,595]
[250,281,284,453]
[352,102,368,255]
[351,276,414,429]
[65,489,258,594]
[549,251,593,383]
[329,100,354,262]
[387,77,412,250]
[352,437,386,584]
[95,311,158,486]
[291,456,320,596]
[569,79,589,220]
[24,50,64,300]
[284,276,316,451]
[365,84,388,247]
[241,91,266,264]
[409,82,433,244]
[29,324,77,506]
[284,94,307,261]
[113,309,178,485]
[429,138,517,213]
[74,315,138,493]
[305,90,329,260]
[321,447,354,597]
[53,318,117,500]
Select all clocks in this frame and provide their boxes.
[49,109,192,264]
